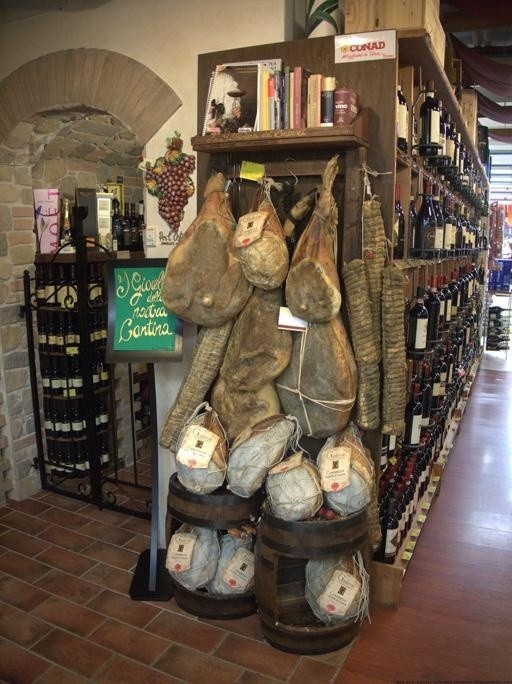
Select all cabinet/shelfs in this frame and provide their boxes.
[190,34,491,611]
[26,251,152,479]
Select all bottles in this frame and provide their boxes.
[60,198,73,247]
[488,305,511,351]
[375,78,492,564]
[112,200,144,251]
[35,263,110,479]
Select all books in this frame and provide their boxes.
[201,54,361,137]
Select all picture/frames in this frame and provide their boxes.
[104,256,183,364]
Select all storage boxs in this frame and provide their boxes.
[343,0,447,67]
